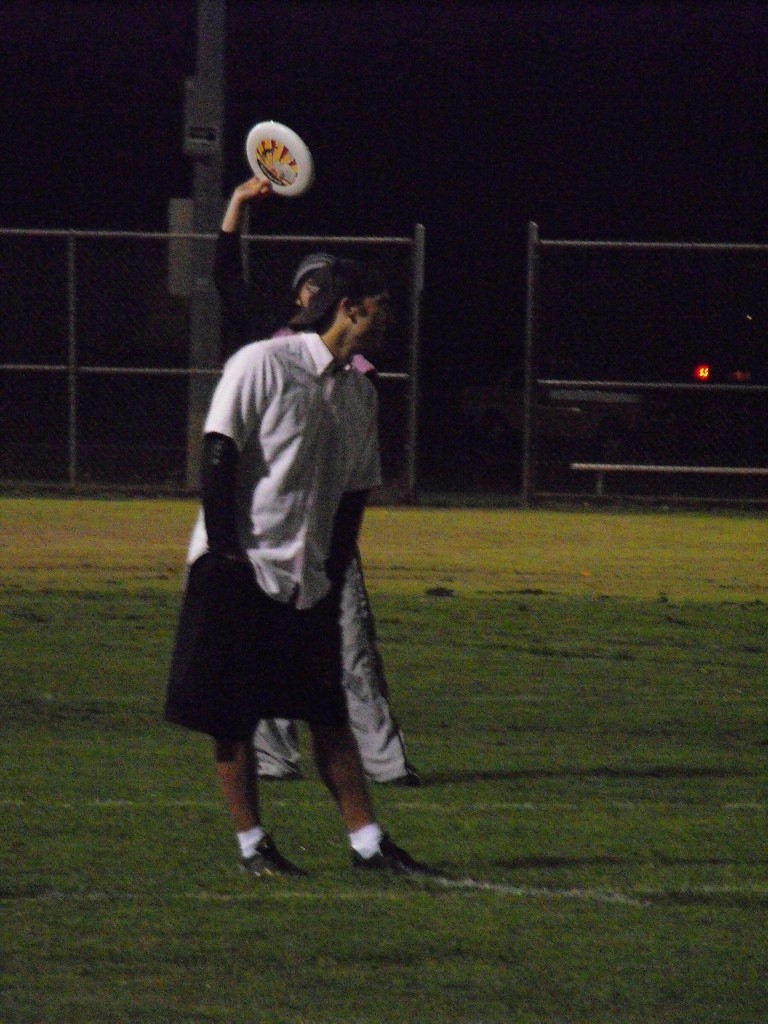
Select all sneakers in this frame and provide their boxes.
[237,837,309,878]
[350,838,450,878]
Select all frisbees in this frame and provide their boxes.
[243,118,316,198]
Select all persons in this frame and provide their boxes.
[211,176,424,786]
[163,261,435,875]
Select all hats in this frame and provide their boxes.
[288,261,381,331]
[293,252,334,287]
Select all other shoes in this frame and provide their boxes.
[375,771,425,787]
[256,770,304,780]
